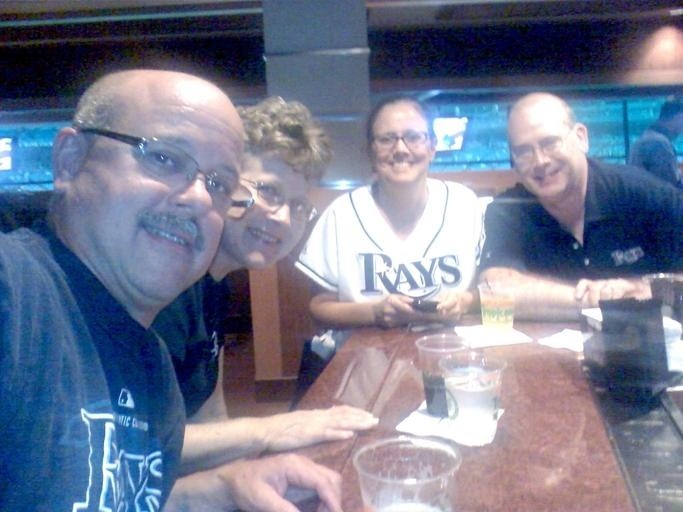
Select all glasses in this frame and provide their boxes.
[369,129,432,148]
[236,175,319,223]
[74,125,255,222]
[510,127,574,164]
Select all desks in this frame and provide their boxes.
[234,295,683,512]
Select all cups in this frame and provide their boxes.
[351,436,461,512]
[479,281,515,328]
[414,334,469,418]
[437,351,508,448]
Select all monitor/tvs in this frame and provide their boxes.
[432,117,467,151]
[0,139,16,174]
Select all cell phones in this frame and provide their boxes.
[411,297,438,311]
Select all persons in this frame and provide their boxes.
[629,98,683,187]
[1,69,343,512]
[148,94,382,473]
[290,96,485,412]
[480,91,683,319]
